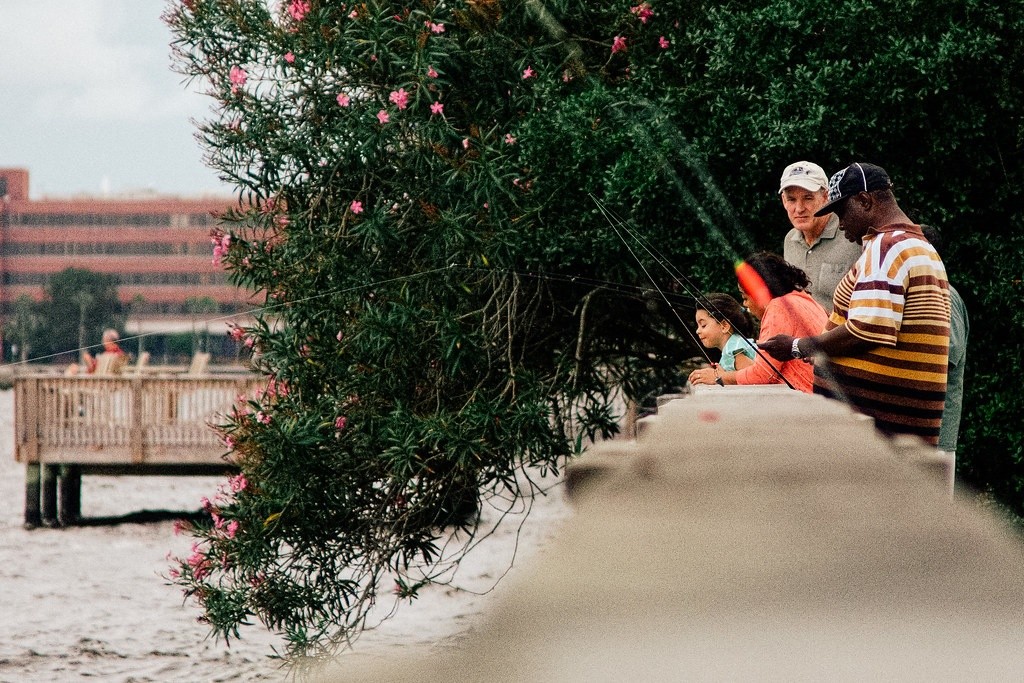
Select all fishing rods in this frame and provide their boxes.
[454,191,795,389]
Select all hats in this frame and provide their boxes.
[778,161,828,195]
[813,163,892,218]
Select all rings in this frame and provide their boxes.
[696,374,700,379]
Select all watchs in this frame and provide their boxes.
[791,338,804,359]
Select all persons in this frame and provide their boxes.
[913,221,970,458]
[83,327,128,375]
[687,250,831,395]
[756,159,953,454]
[777,159,866,317]
[695,292,760,372]
